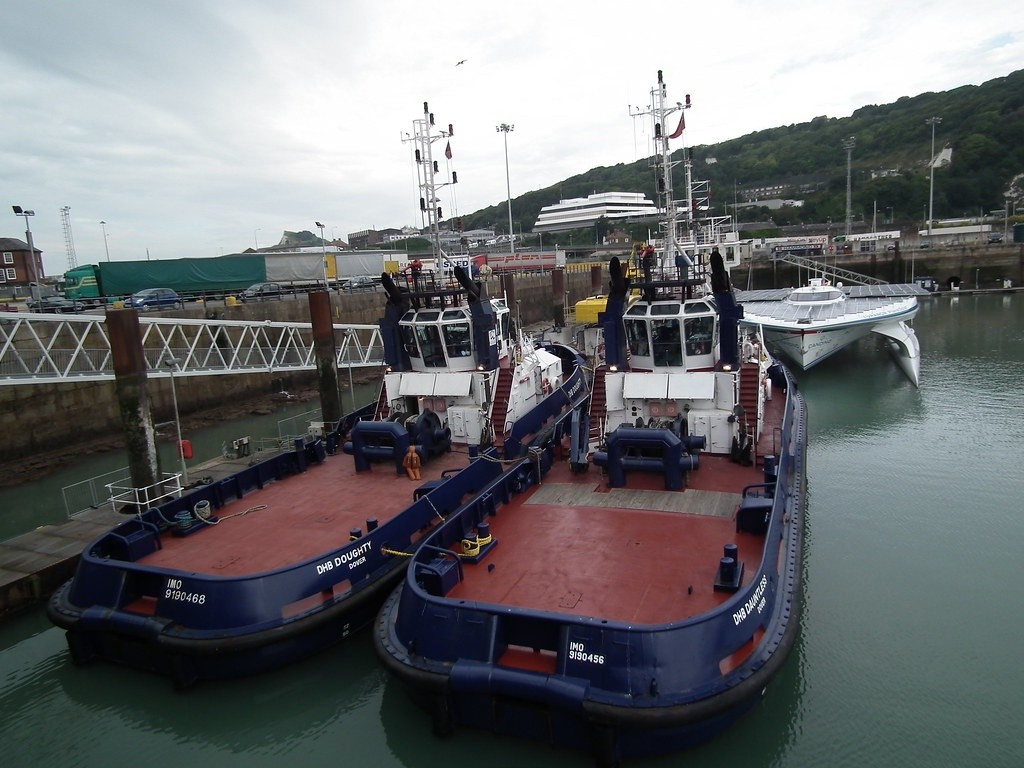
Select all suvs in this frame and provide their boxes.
[239,283,282,302]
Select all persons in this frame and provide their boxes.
[402,446,422,481]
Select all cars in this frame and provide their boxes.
[343,275,374,290]
[988,232,1003,244]
[30,296,85,315]
[125,288,180,311]
[921,240,930,248]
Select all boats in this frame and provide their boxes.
[43,98,591,683]
[373,64,807,758]
[732,258,920,390]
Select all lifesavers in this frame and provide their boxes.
[745,343,753,359]
[598,342,606,360]
[829,246,834,250]
[542,378,549,393]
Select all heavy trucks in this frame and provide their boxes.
[64,253,266,306]
[470,250,565,274]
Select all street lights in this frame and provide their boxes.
[12,205,44,313]
[496,123,514,253]
[315,221,329,292]
[925,115,942,229]
[842,136,856,235]
[100,221,111,262]
